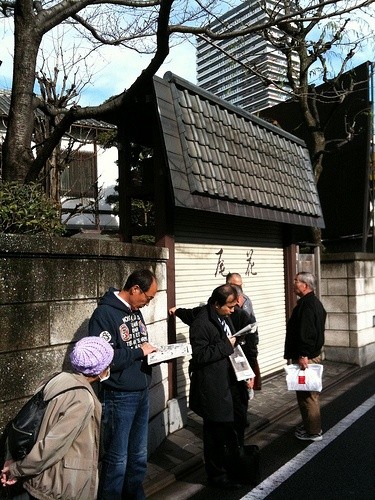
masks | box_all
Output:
[100,366,111,382]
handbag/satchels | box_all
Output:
[283,364,323,392]
[222,425,260,486]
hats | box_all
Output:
[70,336,114,375]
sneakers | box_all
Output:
[295,431,323,441]
[296,424,323,435]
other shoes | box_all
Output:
[208,464,241,489]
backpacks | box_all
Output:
[7,370,92,462]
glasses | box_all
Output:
[107,363,115,368]
[140,288,152,302]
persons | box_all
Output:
[226,272,256,329]
[169,284,261,455]
[88,269,158,500]
[188,284,256,487]
[0,336,114,500]
[283,272,327,441]
